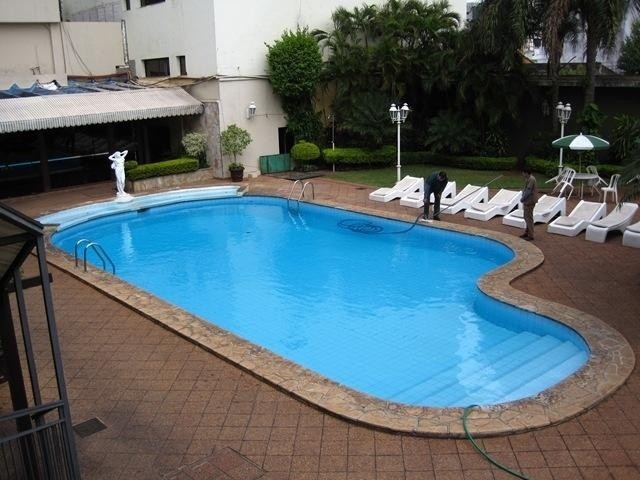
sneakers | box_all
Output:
[422,214,440,220]
[519,233,534,241]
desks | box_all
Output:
[566,173,599,199]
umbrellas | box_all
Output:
[552,130,610,200]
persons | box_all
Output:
[520,166,539,241]
[107,148,132,197]
[421,171,450,223]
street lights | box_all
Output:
[388,102,410,167]
[555,101,572,167]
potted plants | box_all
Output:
[218,124,252,181]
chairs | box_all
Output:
[544,166,568,195]
[586,166,608,196]
[503,194,567,228]
[370,175,424,204]
[601,173,620,204]
[464,188,524,222]
[553,167,576,200]
[586,202,638,243]
[433,184,489,215]
[546,199,607,237]
[622,221,640,248]
[400,181,456,208]
